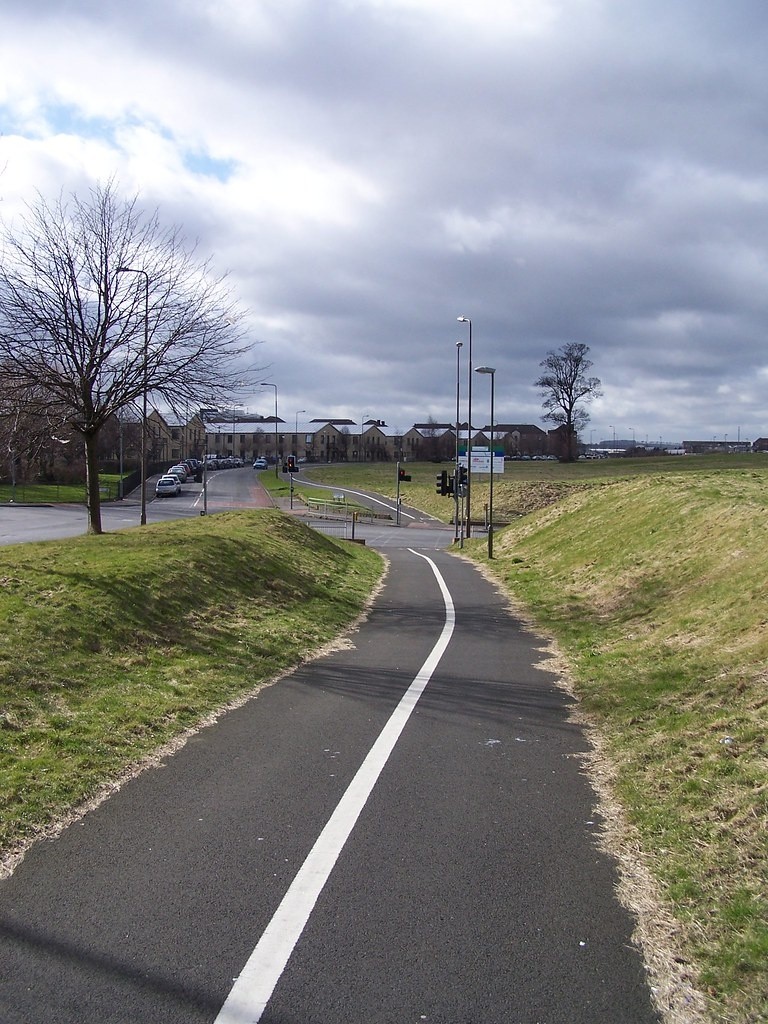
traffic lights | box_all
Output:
[435,470,448,497]
[288,456,300,473]
[398,469,411,482]
[457,465,468,485]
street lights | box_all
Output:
[456,316,472,539]
[725,434,727,451]
[115,265,148,525]
[232,403,244,458]
[629,427,635,448]
[609,426,615,449]
[295,410,306,463]
[455,341,464,538]
[259,383,279,479]
[590,429,596,444]
[361,414,369,434]
[474,366,496,559]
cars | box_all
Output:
[504,454,557,461]
[578,452,610,460]
[155,454,276,498]
[253,459,268,470]
[298,456,308,463]
